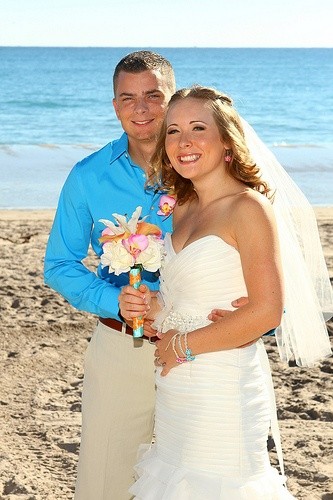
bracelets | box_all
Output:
[165,333,195,363]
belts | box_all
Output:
[99,317,160,344]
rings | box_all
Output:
[157,357,163,366]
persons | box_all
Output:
[44,50,285,500]
[119,87,333,500]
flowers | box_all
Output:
[98,206,166,338]
[157,192,178,222]
